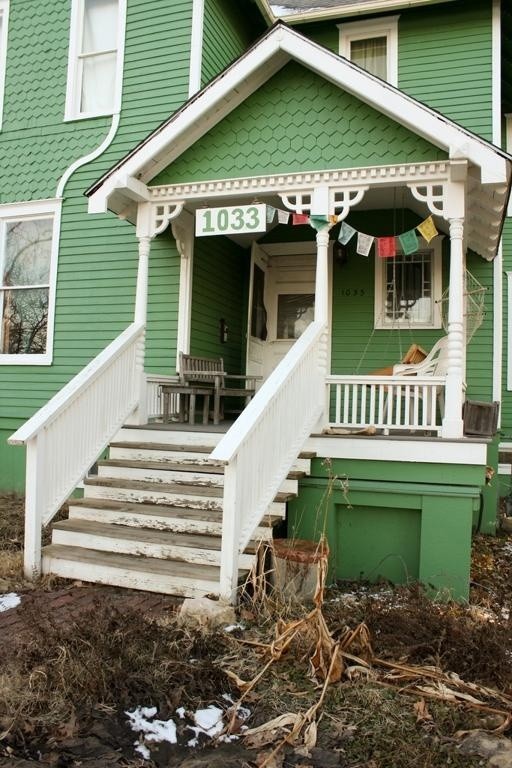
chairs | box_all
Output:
[179,350,262,427]
[382,335,446,435]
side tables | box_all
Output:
[159,384,214,426]
